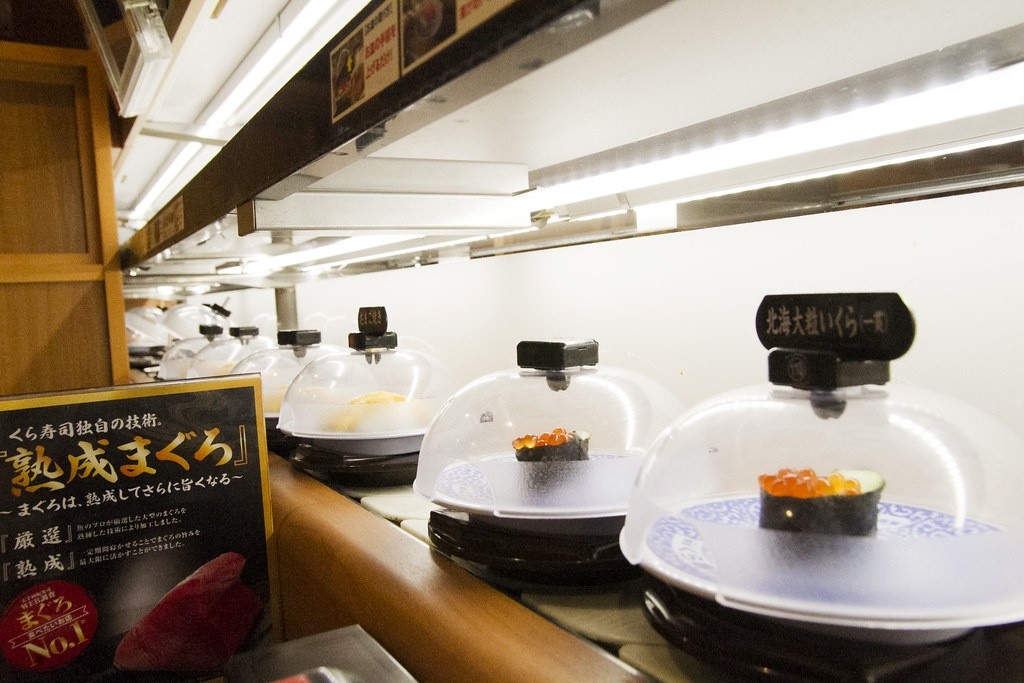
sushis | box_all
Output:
[513,431,590,464]
[761,468,885,533]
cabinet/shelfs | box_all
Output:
[116,0,1024,683]
